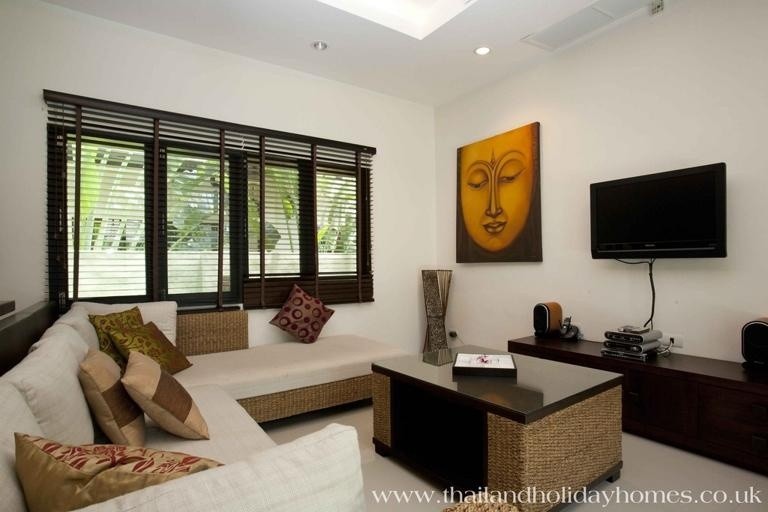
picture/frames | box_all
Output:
[456,121,544,264]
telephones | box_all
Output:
[557,316,579,342]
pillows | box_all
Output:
[268,284,336,343]
[78,346,147,446]
[87,306,146,371]
[119,349,210,440]
[13,432,224,511]
[106,321,191,377]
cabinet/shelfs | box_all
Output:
[506,334,768,477]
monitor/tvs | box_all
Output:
[589,162,728,259]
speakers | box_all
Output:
[533,301,562,338]
[740,317,768,370]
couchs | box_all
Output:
[0,301,396,512]
